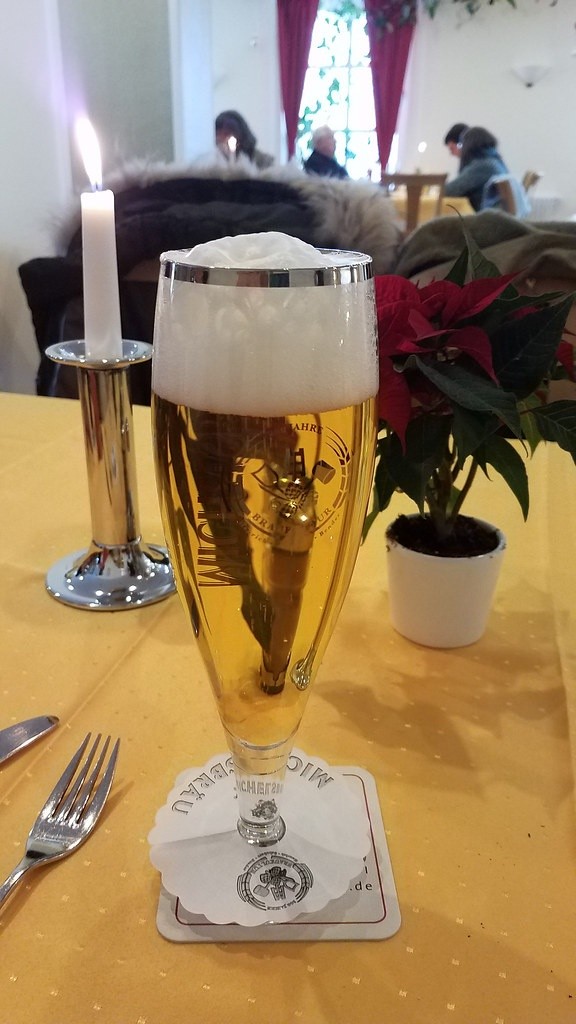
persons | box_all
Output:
[214,108,274,171]
[303,126,354,182]
[441,126,532,220]
[443,122,473,155]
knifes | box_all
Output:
[0,714,60,763]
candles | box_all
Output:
[78,184,131,365]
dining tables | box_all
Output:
[0,383,576,1022]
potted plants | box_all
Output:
[357,199,576,653]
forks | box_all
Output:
[0,732,120,911]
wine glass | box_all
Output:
[145,247,381,925]
